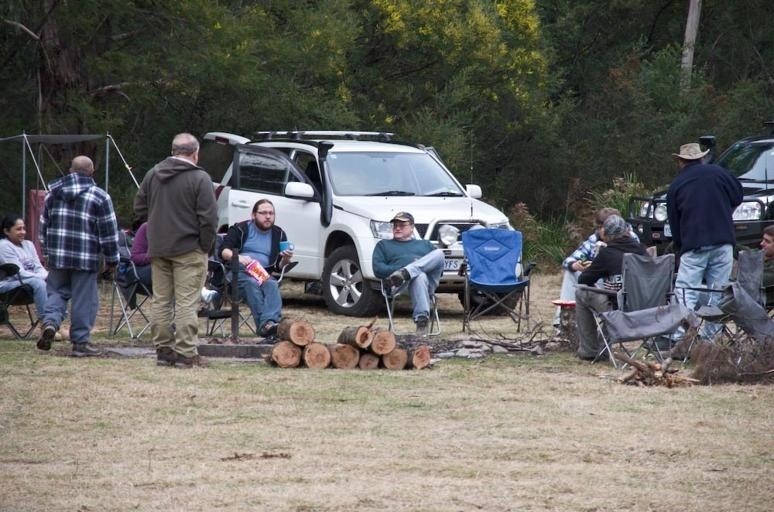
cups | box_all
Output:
[280,241,295,253]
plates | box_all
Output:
[550,299,576,308]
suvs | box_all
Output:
[624,121,774,261]
[196,124,524,318]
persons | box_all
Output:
[0,214,70,342]
[130,221,220,305]
[219,198,295,333]
[553,206,774,360]
[370,210,447,335]
[35,155,122,357]
[133,131,217,369]
[664,142,744,344]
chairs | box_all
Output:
[205,232,299,339]
[108,231,153,339]
[0,263,42,341]
[196,260,231,338]
[380,278,442,338]
[677,251,765,366]
[462,228,530,332]
[574,254,676,369]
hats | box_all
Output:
[671,143,710,161]
[603,215,625,234]
[390,212,414,224]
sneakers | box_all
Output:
[157,350,209,369]
[416,316,428,339]
[72,342,102,356]
[202,287,218,304]
[659,346,690,359]
[383,271,404,290]
[37,325,62,349]
[259,321,279,337]
